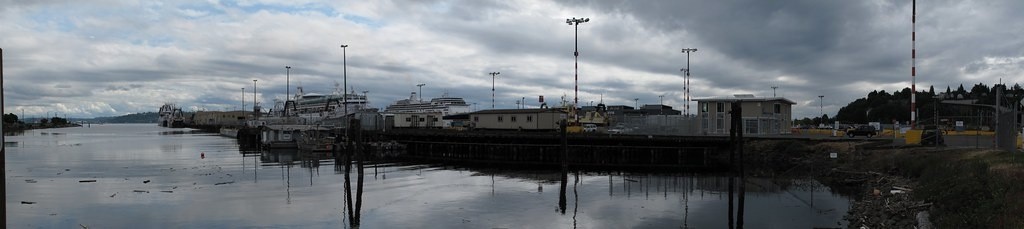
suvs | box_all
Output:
[847,125,877,138]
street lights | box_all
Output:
[252,78,258,113]
[817,95,824,123]
[417,83,425,111]
[681,48,697,118]
[659,94,665,115]
[679,66,688,116]
[285,66,291,115]
[489,71,500,109]
[566,16,589,110]
[771,85,778,98]
[241,87,247,113]
[340,43,349,118]
[634,97,639,113]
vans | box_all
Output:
[583,124,598,133]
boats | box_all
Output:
[158,78,379,154]
